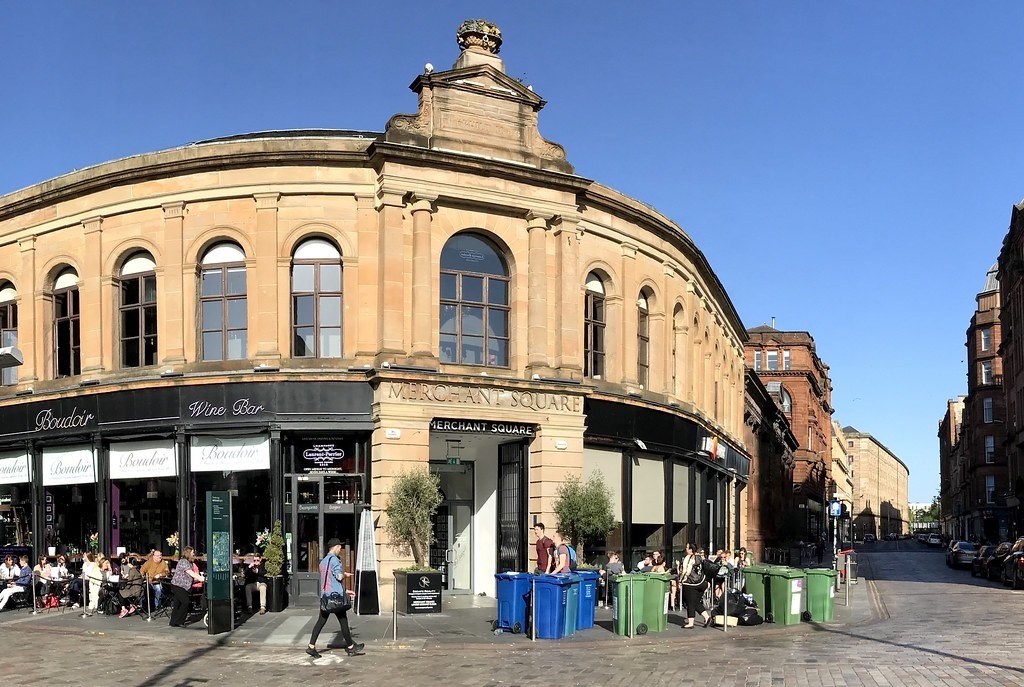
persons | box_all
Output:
[592,540,751,629]
[305,538,365,657]
[0,547,267,628]
[815,535,828,566]
[534,523,556,575]
[550,529,572,573]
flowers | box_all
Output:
[256,527,272,548]
[89,532,99,549]
[166,531,179,547]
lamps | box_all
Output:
[365,368,376,378]
[633,438,647,450]
[698,451,710,457]
[442,304,470,320]
[531,373,541,380]
[424,62,434,75]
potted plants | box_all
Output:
[45,528,57,556]
[382,465,444,617]
[549,465,624,609]
[264,520,285,612]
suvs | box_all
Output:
[863,534,874,544]
[986,541,1013,579]
[971,545,998,577]
[1000,536,1024,590]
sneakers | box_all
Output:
[306,647,321,658]
[348,642,364,655]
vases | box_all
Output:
[92,548,99,555]
[174,545,179,557]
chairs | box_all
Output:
[0,558,264,624]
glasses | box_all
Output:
[739,553,745,555]
[721,556,726,559]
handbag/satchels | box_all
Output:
[46,593,60,608]
[702,559,720,581]
[321,593,352,612]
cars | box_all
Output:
[883,533,979,569]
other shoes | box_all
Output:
[129,605,137,613]
[260,608,265,615]
[119,609,128,618]
[704,618,712,628]
[681,623,694,629]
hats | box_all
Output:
[328,538,345,547]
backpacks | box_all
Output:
[560,543,577,570]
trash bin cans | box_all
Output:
[556,572,584,637]
[640,571,675,632]
[803,567,838,623]
[492,572,533,634]
[609,573,651,636]
[743,565,771,623]
[766,565,806,626]
[570,569,601,631]
[734,550,753,565]
[528,573,573,639]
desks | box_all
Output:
[228,338,242,359]
